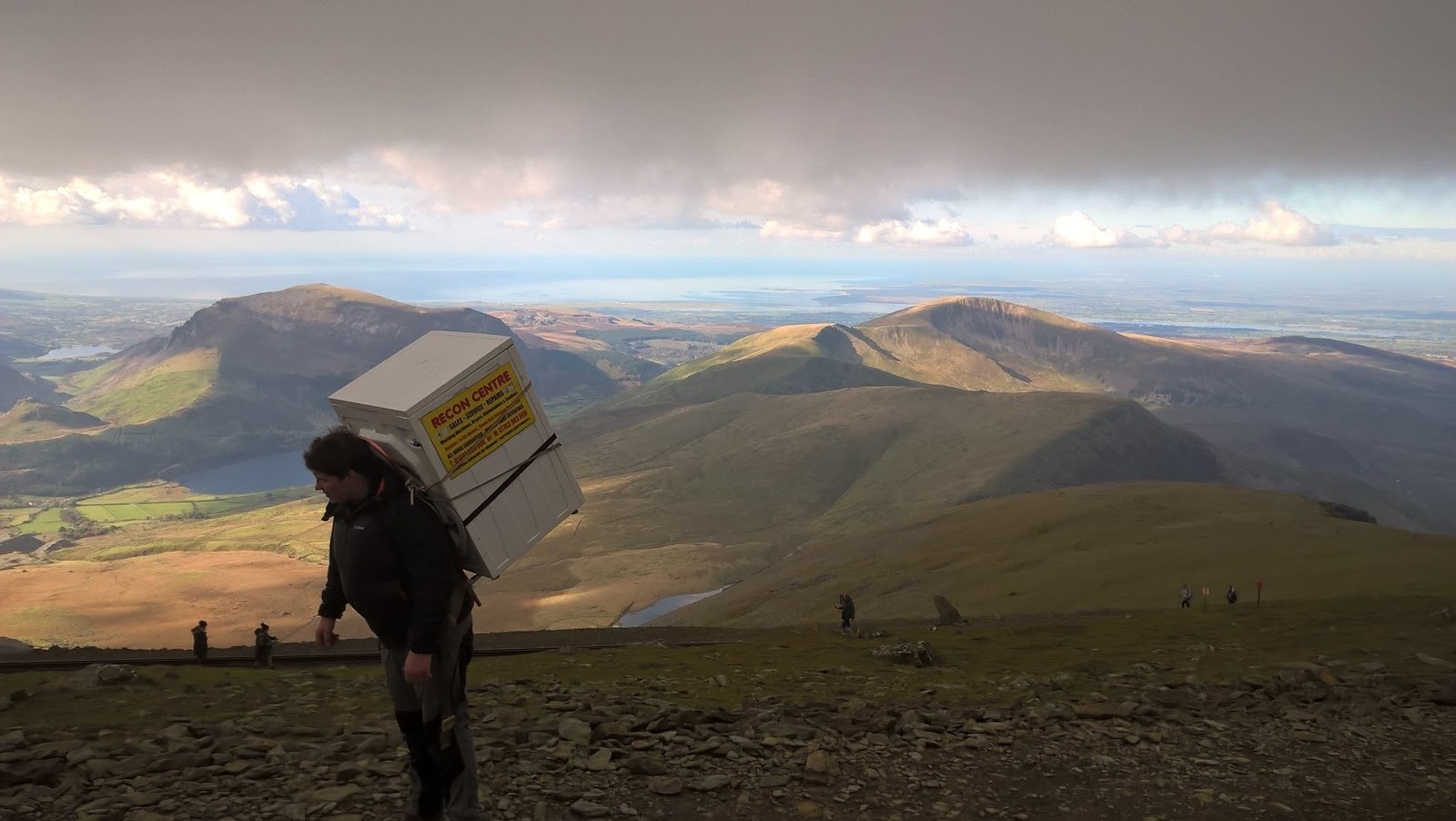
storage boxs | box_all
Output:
[330,329,586,582]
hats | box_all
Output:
[199,621,207,626]
[261,623,269,630]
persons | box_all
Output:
[254,623,279,669]
[925,593,968,632]
[1178,584,1192,609]
[835,594,854,629]
[301,426,482,821]
[191,620,208,665]
[1226,586,1237,604]
